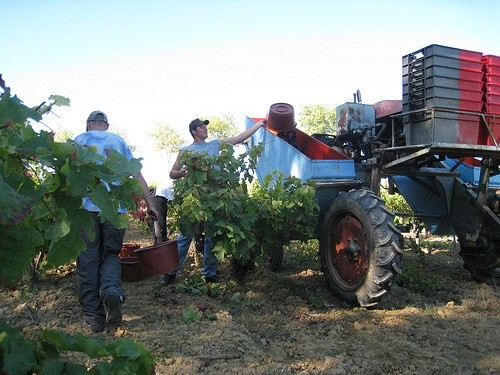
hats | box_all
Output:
[189,118,209,132]
[87,111,107,131]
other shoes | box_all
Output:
[159,276,174,287]
[104,293,122,326]
[206,275,223,283]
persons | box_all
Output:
[159,118,266,285]
[149,186,175,244]
[74,111,159,332]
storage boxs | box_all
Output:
[402,44,500,145]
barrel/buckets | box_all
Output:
[133,214,180,276]
[119,257,143,282]
[266,103,297,133]
[119,244,140,258]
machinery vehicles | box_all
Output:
[239,89,500,309]
